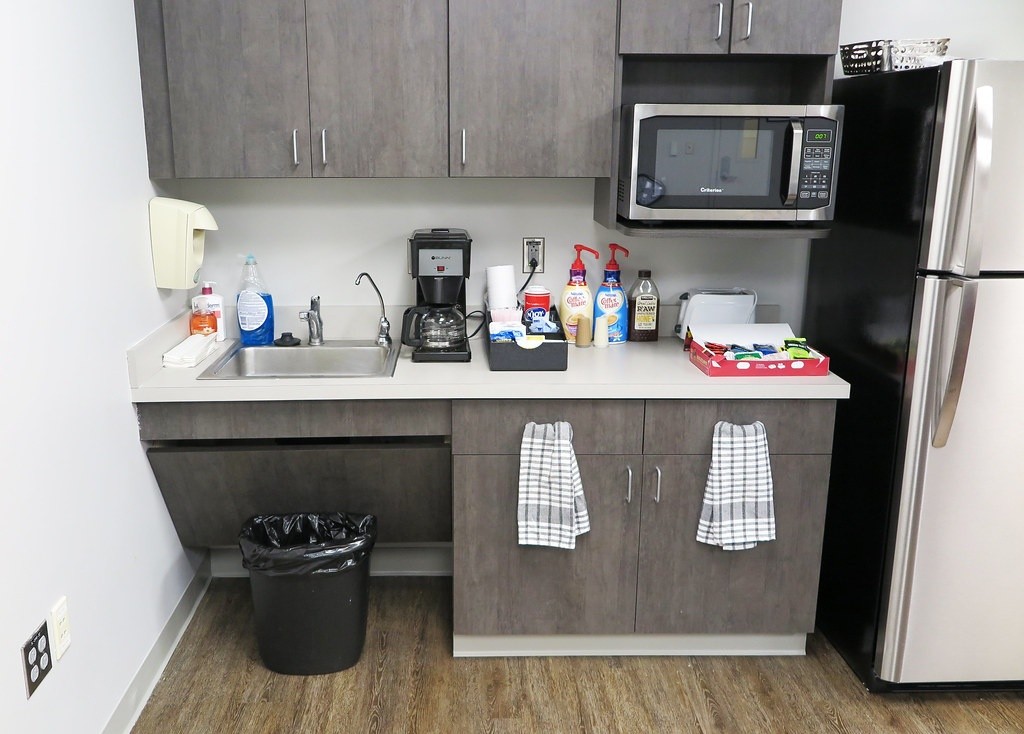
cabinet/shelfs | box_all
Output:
[450,0,623,178]
[161,0,448,179]
[619,0,843,105]
[451,398,837,654]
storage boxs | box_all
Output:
[683,324,829,377]
[486,301,568,373]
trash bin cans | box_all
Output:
[238,514,378,676]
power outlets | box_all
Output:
[50,596,71,660]
[21,619,53,700]
[522,237,545,273]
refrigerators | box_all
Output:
[800,55,1024,695]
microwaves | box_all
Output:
[619,104,848,229]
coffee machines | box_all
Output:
[401,228,472,363]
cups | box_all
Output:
[575,318,592,348]
[593,317,609,349]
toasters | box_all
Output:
[674,286,757,340]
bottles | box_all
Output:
[236,255,275,347]
[627,269,661,342]
